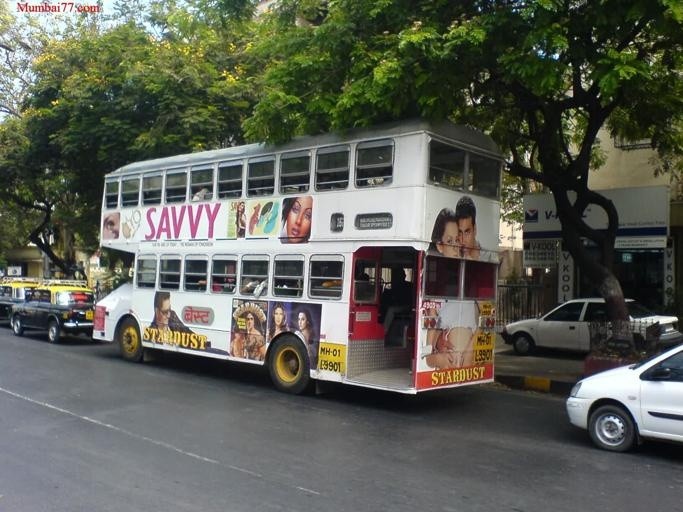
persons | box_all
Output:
[292,306,318,357]
[233,202,246,237]
[106,213,120,235]
[423,300,485,374]
[233,312,265,362]
[382,266,413,341]
[269,303,291,349]
[280,196,311,243]
[191,176,213,201]
[146,289,229,356]
[197,259,300,299]
[456,195,498,264]
[428,208,460,257]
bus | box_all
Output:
[88,114,509,405]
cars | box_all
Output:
[498,292,682,359]
[563,341,682,455]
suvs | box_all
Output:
[0,274,37,322]
[9,279,96,344]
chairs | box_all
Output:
[380,281,418,347]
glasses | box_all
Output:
[161,305,172,315]
